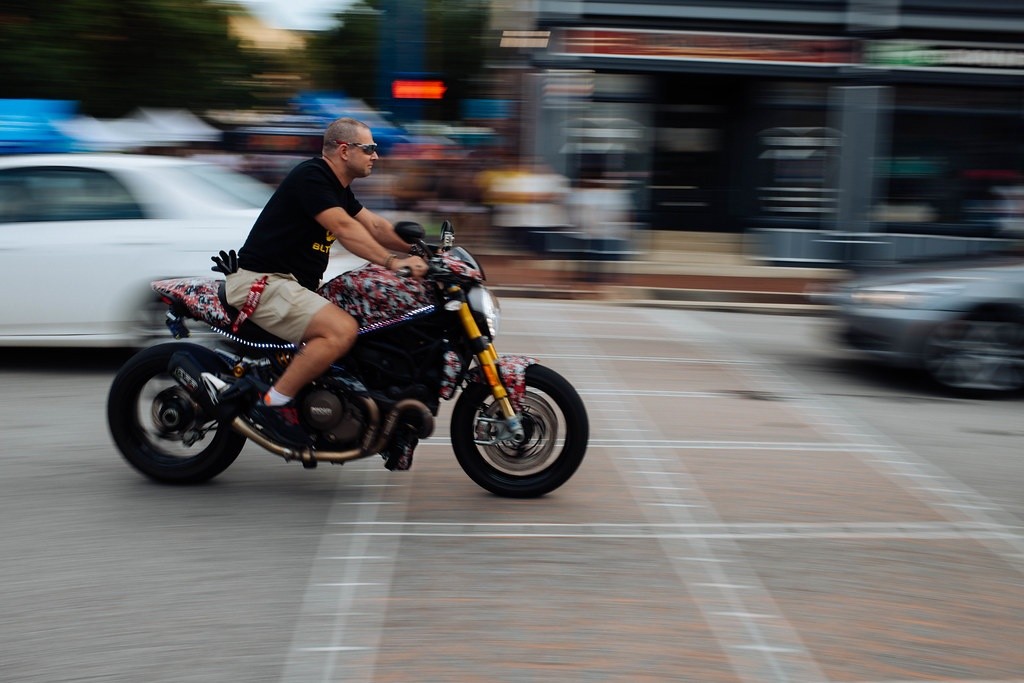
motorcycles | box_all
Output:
[107,219,589,497]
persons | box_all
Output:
[227,118,429,455]
[475,154,632,261]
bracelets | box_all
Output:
[385,255,396,269]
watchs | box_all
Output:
[411,244,418,253]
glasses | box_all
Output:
[335,141,378,155]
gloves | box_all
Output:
[211,250,239,275]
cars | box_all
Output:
[830,252,1024,397]
[0,153,369,347]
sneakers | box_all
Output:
[248,395,307,449]
